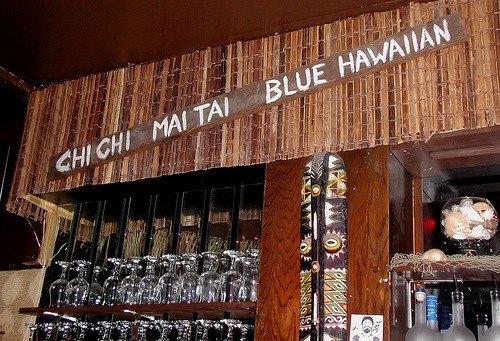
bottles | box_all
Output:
[404,288,436,341]
[426,289,443,341]
[441,291,477,341]
[478,289,500,341]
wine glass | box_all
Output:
[49,261,70,307]
[24,317,254,341]
[63,248,260,303]
